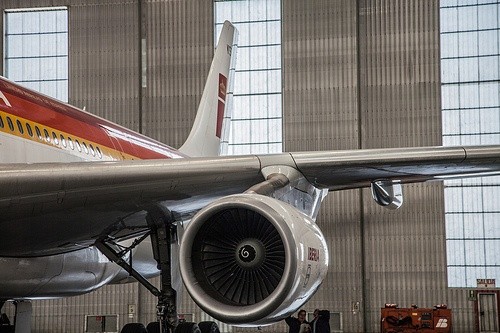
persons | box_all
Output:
[284,309,311,333]
[309,308,332,332]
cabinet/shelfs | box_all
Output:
[381,308,452,333]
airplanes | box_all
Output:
[1,20,500,332]
